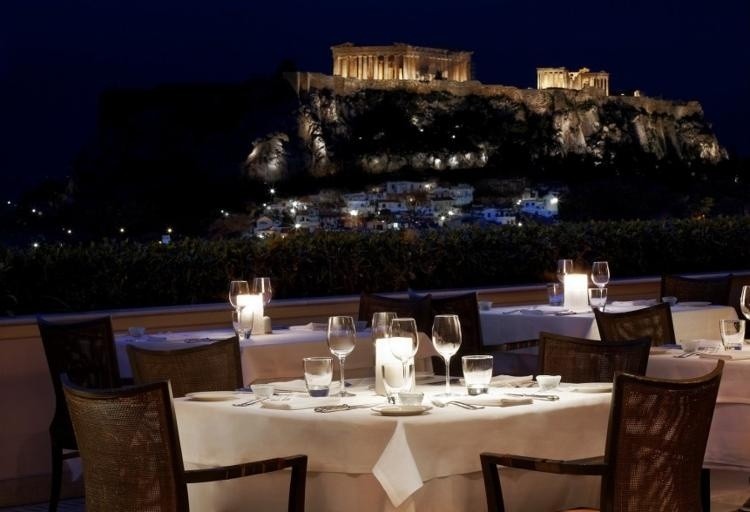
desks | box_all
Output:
[116,321,439,376]
[480,297,648,373]
[168,375,618,512]
[650,340,750,476]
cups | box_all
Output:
[251,383,274,398]
[536,374,561,391]
[719,319,745,351]
[381,364,414,404]
[740,285,750,320]
[461,354,493,397]
[303,357,332,396]
[228,276,273,338]
[544,259,611,315]
[663,296,677,306]
[397,391,424,405]
[372,313,399,348]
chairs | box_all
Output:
[659,271,737,304]
[39,314,132,509]
[594,301,679,347]
[125,335,245,395]
[60,374,308,512]
[535,332,653,382]
[405,288,494,376]
[479,359,732,511]
[355,292,434,331]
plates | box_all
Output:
[186,390,240,399]
[678,302,712,306]
[372,404,434,415]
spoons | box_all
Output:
[432,397,484,409]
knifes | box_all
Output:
[316,403,376,414]
[232,397,268,406]
[507,393,559,400]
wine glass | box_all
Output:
[387,319,420,378]
[327,315,358,397]
[432,315,463,397]
